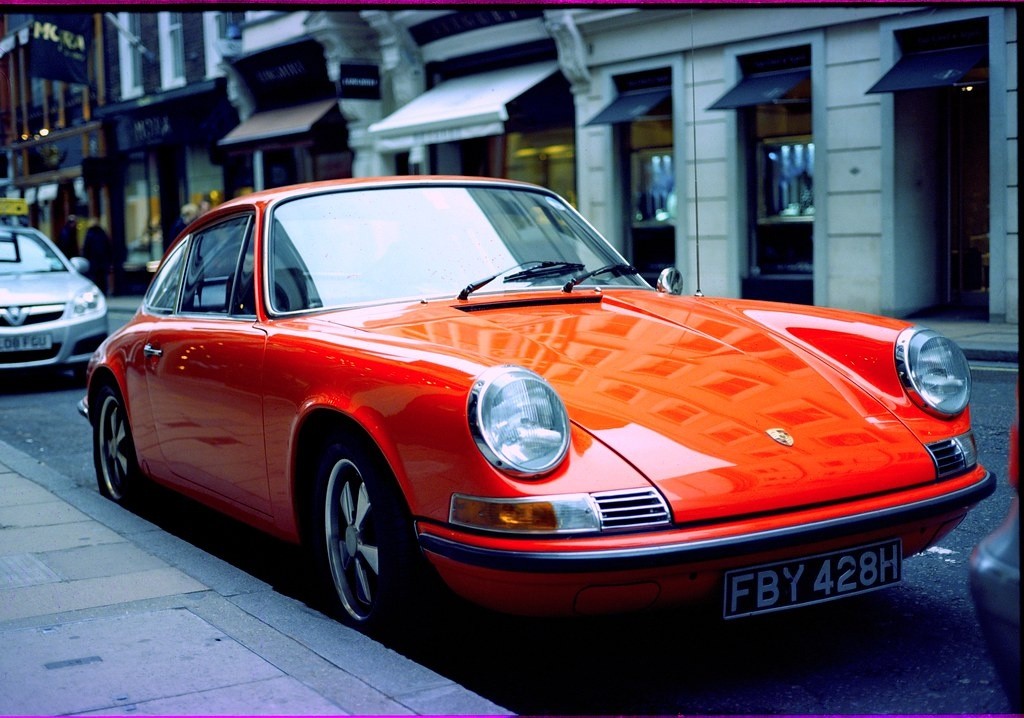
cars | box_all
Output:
[0,225,109,392]
[75,174,996,647]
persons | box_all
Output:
[59,214,110,298]
